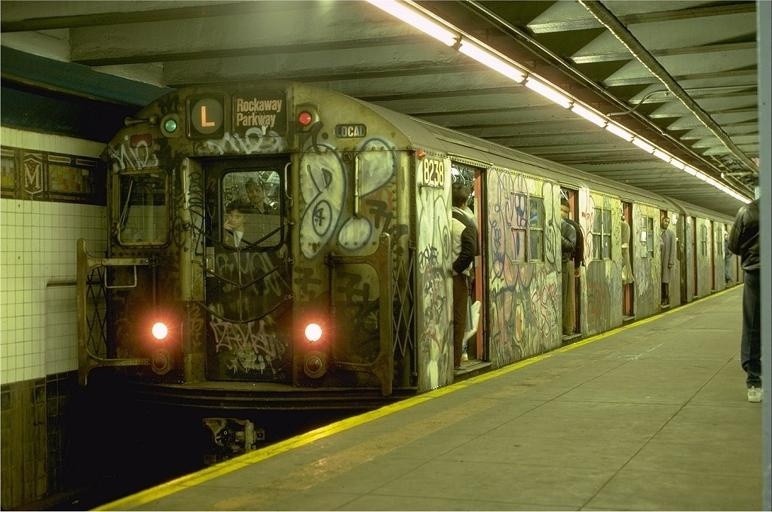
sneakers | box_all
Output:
[747,386,762,402]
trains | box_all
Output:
[75,77,743,466]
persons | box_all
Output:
[659,216,675,308]
[222,198,266,247]
[561,198,576,338]
[725,232,733,288]
[245,179,280,247]
[451,182,480,370]
[727,195,764,401]
[563,216,583,330]
